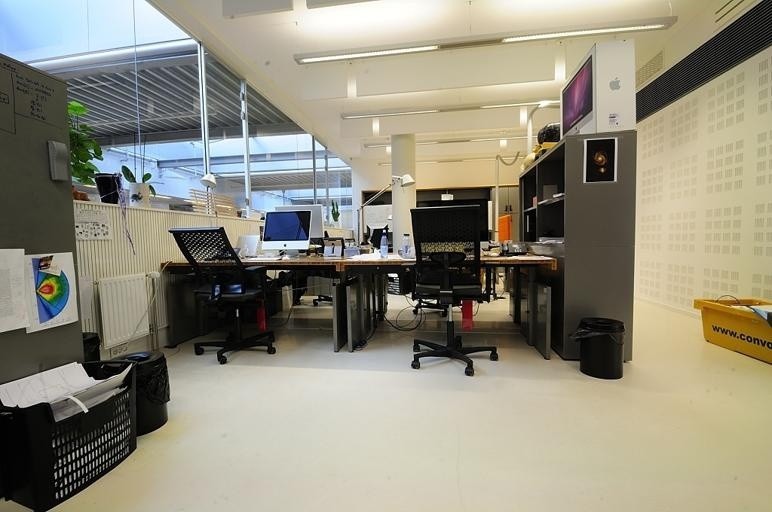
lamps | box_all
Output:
[200,173,223,227]
[352,174,415,253]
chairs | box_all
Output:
[167,226,282,366]
[410,205,500,379]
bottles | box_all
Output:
[380,229,388,260]
[402,233,411,260]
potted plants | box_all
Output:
[329,200,341,227]
[120,165,167,207]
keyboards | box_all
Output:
[248,256,281,261]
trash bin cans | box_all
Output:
[104,350,171,439]
[567,316,626,380]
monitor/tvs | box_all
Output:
[274,204,325,249]
[261,210,313,261]
[559,44,595,139]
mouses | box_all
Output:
[283,255,290,261]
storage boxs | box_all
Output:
[693,296,771,365]
[0,359,137,511]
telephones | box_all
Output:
[502,242,528,257]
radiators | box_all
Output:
[96,273,152,348]
[153,268,166,328]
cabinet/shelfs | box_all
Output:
[520,129,635,362]
[493,187,519,243]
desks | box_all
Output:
[346,248,558,362]
[162,250,344,352]
